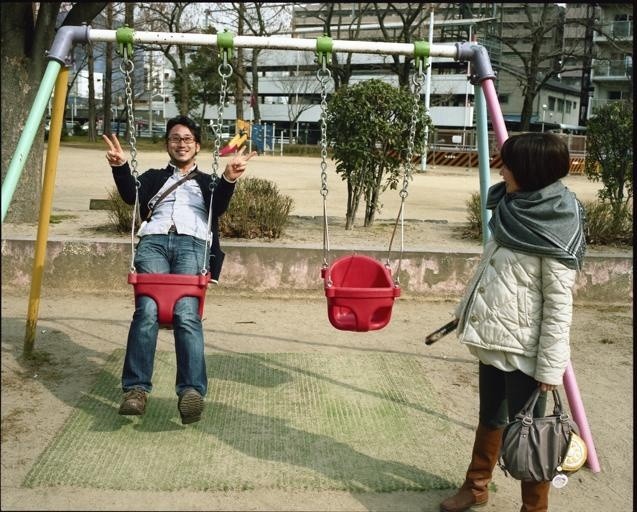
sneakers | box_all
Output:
[178,388,205,426]
[118,380,150,416]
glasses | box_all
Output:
[169,136,195,144]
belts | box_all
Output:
[168,225,179,234]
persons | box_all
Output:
[102,116,256,425]
[440,133,585,512]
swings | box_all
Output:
[311,51,429,333]
[115,41,232,331]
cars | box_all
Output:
[44,119,307,143]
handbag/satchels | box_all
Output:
[500,416,582,481]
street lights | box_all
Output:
[540,104,547,133]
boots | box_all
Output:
[440,422,509,511]
[520,478,549,512]
